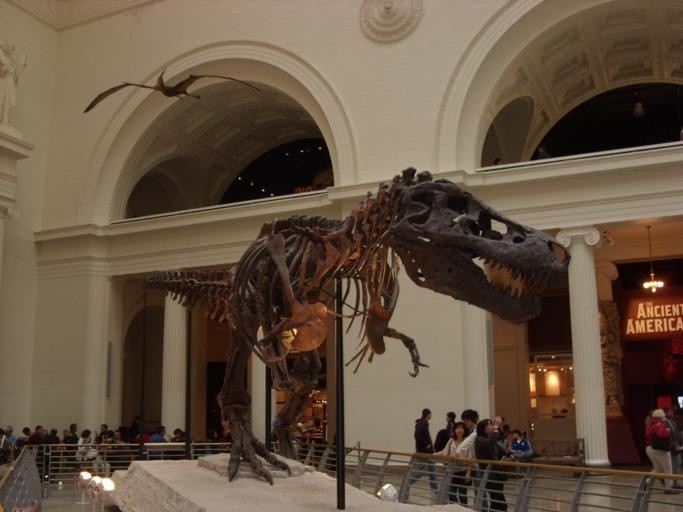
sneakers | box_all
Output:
[664,484,683,494]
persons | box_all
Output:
[642,406,683,495]
[404,406,535,512]
[0,414,232,487]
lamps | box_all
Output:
[643,225,664,293]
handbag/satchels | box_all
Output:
[651,422,669,451]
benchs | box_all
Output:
[529,438,587,478]
